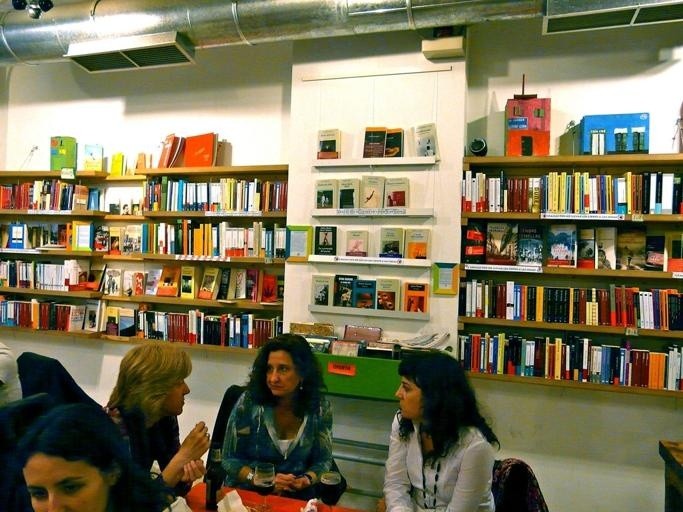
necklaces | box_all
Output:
[418,422,441,509]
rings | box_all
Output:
[203,432,209,439]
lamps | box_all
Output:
[11,0,54,18]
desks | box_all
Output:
[658,439,683,512]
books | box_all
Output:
[141,218,285,259]
[363,127,404,157]
[109,225,143,254]
[314,226,337,256]
[0,294,108,332]
[105,306,137,337]
[457,333,681,391]
[137,309,283,349]
[314,176,410,210]
[461,219,681,272]
[380,228,406,258]
[145,263,285,302]
[310,274,429,314]
[461,170,681,214]
[290,323,454,360]
[157,134,232,167]
[415,123,442,162]
[0,255,107,292]
[458,279,681,331]
[0,178,106,211]
[404,229,430,259]
[105,269,144,296]
[49,136,152,177]
[345,230,369,257]
[318,129,342,158]
[144,175,288,212]
[0,219,108,252]
[107,199,142,215]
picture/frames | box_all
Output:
[431,262,458,296]
[285,225,312,262]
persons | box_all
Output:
[380,344,501,511]
[0,342,22,413]
[219,333,335,505]
[0,401,167,511]
[99,339,211,509]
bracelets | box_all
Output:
[245,469,256,484]
[296,472,312,486]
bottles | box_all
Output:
[206,442,226,510]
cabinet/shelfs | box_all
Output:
[308,154,435,320]
[133,163,289,354]
[96,174,147,342]
[0,170,107,339]
[308,352,406,400]
[457,151,683,397]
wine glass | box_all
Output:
[320,471,342,512]
[255,463,276,511]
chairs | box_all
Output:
[203,383,347,506]
[0,351,103,512]
[487,454,549,512]
